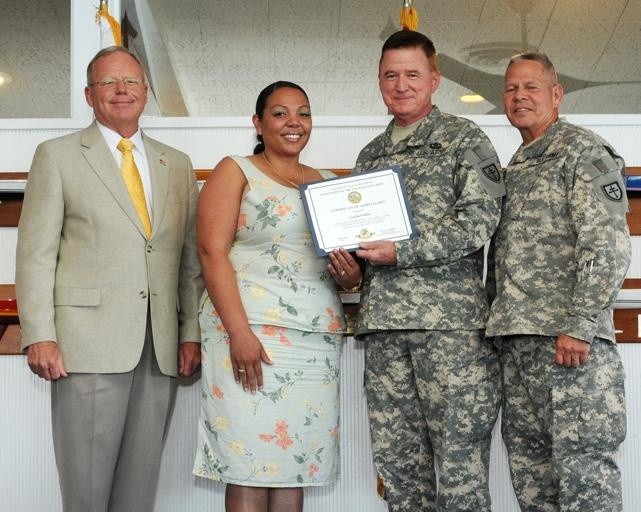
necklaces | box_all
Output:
[262,150,304,189]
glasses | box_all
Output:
[90,80,144,87]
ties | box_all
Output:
[117,139,152,239]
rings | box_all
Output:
[341,270,345,275]
[238,369,245,372]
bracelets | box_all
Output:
[341,279,362,294]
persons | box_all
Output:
[192,79,364,512]
[14,43,210,512]
[352,28,509,512]
[484,51,633,512]
